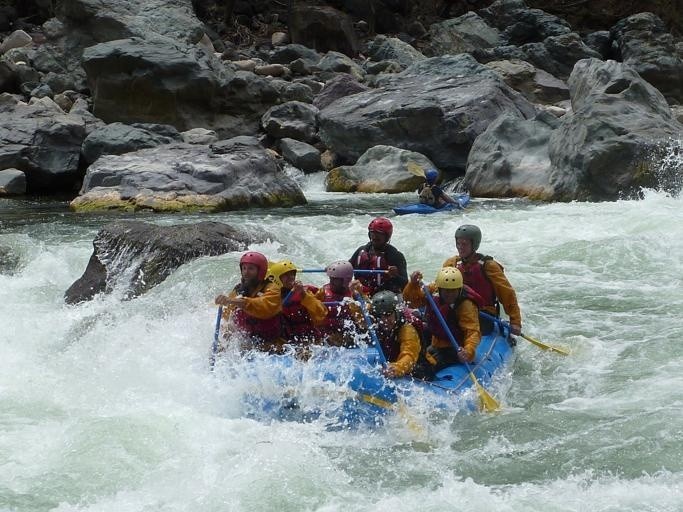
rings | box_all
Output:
[462,356,464,359]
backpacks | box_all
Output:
[417,182,436,206]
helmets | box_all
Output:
[424,168,438,179]
[368,218,393,244]
[369,290,399,315]
[324,260,353,289]
[267,259,298,290]
[455,224,482,253]
[434,265,464,291]
[239,251,267,283]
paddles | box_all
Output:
[354,290,421,435]
[266,260,390,274]
[479,309,568,356]
[418,278,499,413]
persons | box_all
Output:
[211,251,281,365]
[441,223,522,337]
[347,214,408,300]
[267,259,329,364]
[363,289,427,383]
[402,266,482,375]
[319,260,368,351]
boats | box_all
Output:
[235,315,514,439]
[394,191,471,216]
